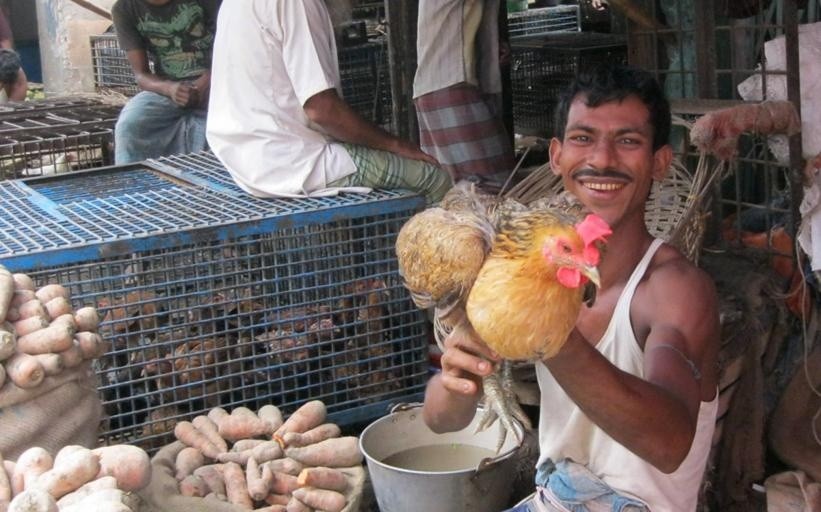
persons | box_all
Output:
[112,0,217,170]
[204,0,450,203]
[421,66,726,512]
[413,0,512,192]
[0,49,27,104]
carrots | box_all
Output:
[1,263,364,512]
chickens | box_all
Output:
[20,151,76,177]
[73,236,427,455]
[392,169,618,454]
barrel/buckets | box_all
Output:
[359,401,524,512]
